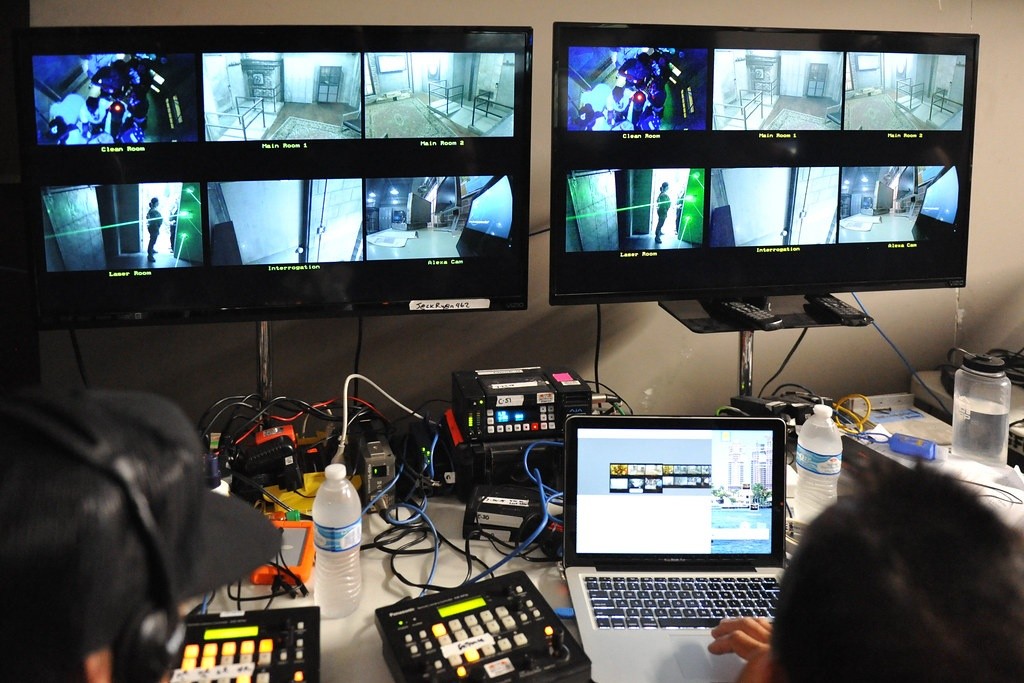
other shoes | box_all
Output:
[655,237,662,242]
[660,232,665,235]
[153,249,158,252]
[148,256,155,261]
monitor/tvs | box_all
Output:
[12,23,981,329]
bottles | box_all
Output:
[312,463,364,619]
[793,404,843,525]
[948,348,1012,468]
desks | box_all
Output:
[911,371,1024,460]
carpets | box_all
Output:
[268,117,342,140]
[765,109,824,130]
[365,96,457,139]
[844,94,917,130]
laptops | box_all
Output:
[561,415,790,683]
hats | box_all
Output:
[0,390,283,669]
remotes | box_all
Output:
[804,294,874,327]
[698,300,783,332]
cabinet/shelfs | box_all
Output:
[317,66,342,104]
[804,62,828,98]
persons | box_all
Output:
[709,468,1024,683]
[169,196,179,252]
[0,390,285,682]
[147,197,163,261]
[655,182,671,243]
[676,184,686,233]
[578,48,673,130]
[49,58,152,144]
